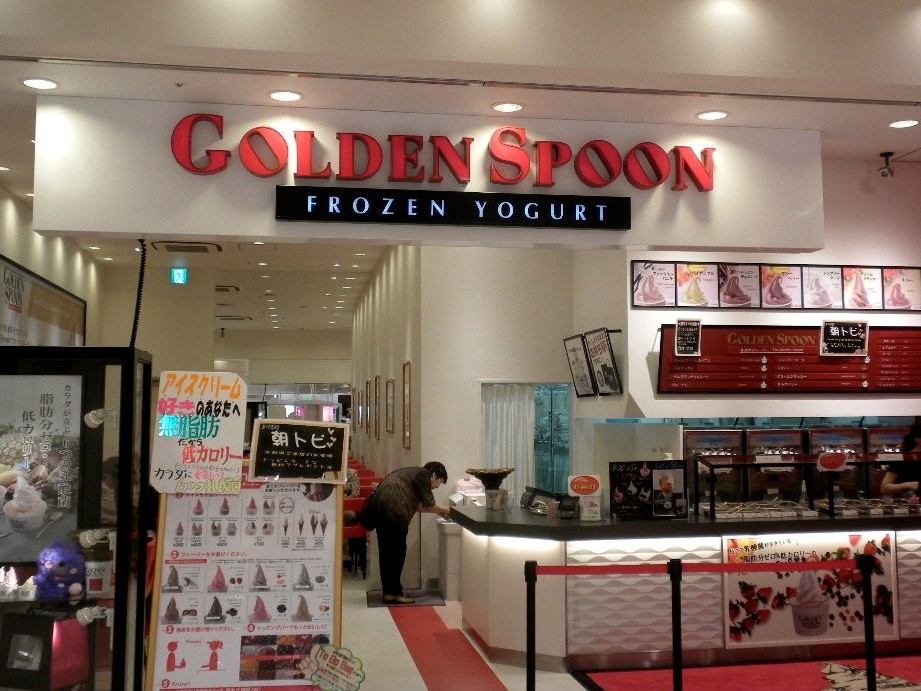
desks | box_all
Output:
[1,598,96,691]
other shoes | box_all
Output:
[383,593,415,604]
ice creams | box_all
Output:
[633,275,666,306]
[2,475,48,532]
[886,280,911,309]
[762,276,793,308]
[806,277,832,308]
[847,271,870,309]
[720,276,752,307]
[789,556,830,637]
[614,460,676,509]
[162,496,328,623]
[678,277,709,306]
[0,566,37,601]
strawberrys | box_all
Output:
[729,580,798,644]
[818,534,891,591]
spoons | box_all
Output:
[31,512,64,540]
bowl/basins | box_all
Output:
[4,498,49,531]
[789,599,831,637]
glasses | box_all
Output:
[436,472,444,486]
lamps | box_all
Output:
[76,604,113,630]
[78,529,116,553]
[83,407,120,433]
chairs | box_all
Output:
[342,455,386,578]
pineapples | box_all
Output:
[0,420,81,469]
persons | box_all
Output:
[879,422,921,495]
[358,460,450,605]
[342,466,361,496]
[101,457,123,525]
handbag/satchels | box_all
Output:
[360,492,382,531]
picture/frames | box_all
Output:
[402,360,412,449]
[356,390,363,428]
[374,375,380,439]
[365,381,371,438]
[351,389,357,433]
[385,380,395,433]
